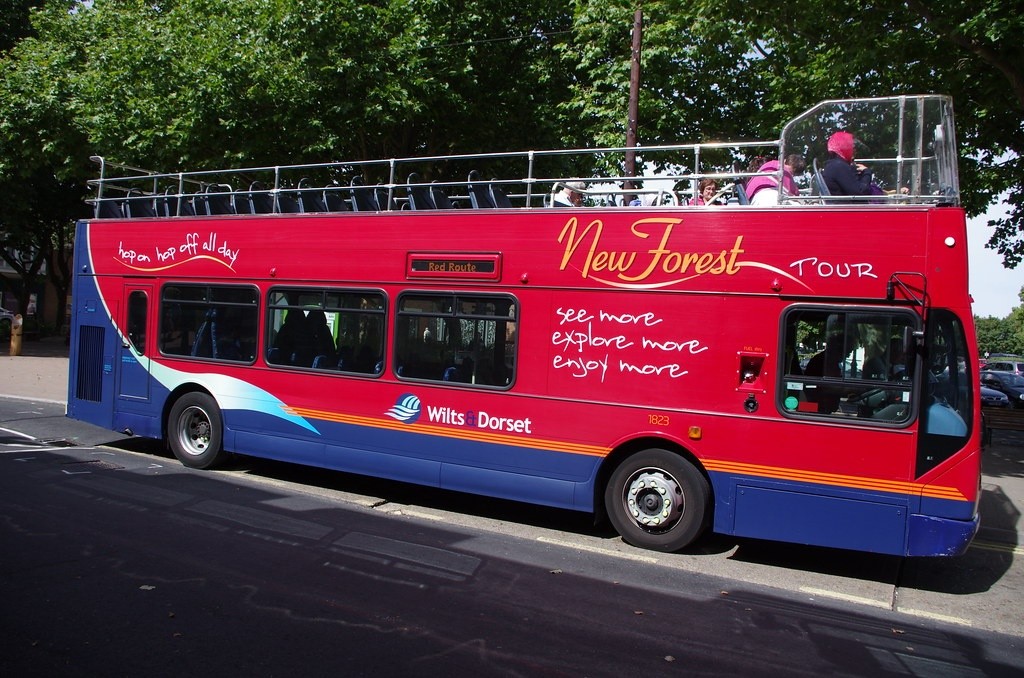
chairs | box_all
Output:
[99,155,844,219]
[126,289,514,388]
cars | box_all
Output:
[797,353,1024,409]
[0,272,71,345]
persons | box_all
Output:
[554,177,586,206]
[747,154,804,207]
[275,311,336,366]
[824,132,909,204]
[803,334,858,414]
[689,179,722,205]
[863,339,905,379]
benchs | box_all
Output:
[980,406,1024,448]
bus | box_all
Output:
[64,94,983,557]
